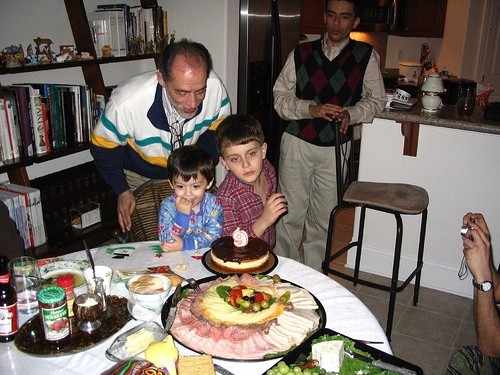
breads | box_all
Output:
[177,355,216,375]
[128,276,165,294]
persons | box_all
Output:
[158,145,224,252]
[129,29,175,56]
[462,212,500,357]
[216,113,287,250]
[273,0,388,270]
[89,37,232,233]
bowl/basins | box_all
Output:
[125,273,173,309]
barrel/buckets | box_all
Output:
[91,4,130,57]
[137,0,167,55]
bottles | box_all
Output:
[73,294,100,333]
[0,256,18,342]
[93,278,107,312]
[57,274,76,319]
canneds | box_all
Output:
[36,286,70,344]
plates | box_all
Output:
[38,261,91,290]
[99,355,172,375]
[259,328,424,375]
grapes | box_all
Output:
[242,300,269,310]
[273,274,280,283]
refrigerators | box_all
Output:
[237,0,304,177]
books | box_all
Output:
[0,83,105,159]
[90,4,167,57]
[0,182,45,248]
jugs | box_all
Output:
[420,71,447,93]
[421,91,444,113]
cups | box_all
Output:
[8,256,41,315]
[84,266,112,296]
[458,86,475,118]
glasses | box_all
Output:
[169,120,183,151]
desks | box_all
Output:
[0,240,393,375]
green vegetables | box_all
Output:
[215,285,276,313]
[263,333,404,375]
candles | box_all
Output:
[232,227,248,247]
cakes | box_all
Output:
[211,228,270,272]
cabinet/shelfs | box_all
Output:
[301,0,328,33]
[0,52,173,278]
[387,0,448,38]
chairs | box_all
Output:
[113,177,219,243]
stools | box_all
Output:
[322,118,429,343]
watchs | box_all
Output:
[472,280,493,292]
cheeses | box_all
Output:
[311,340,344,373]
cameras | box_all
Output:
[461,218,476,243]
